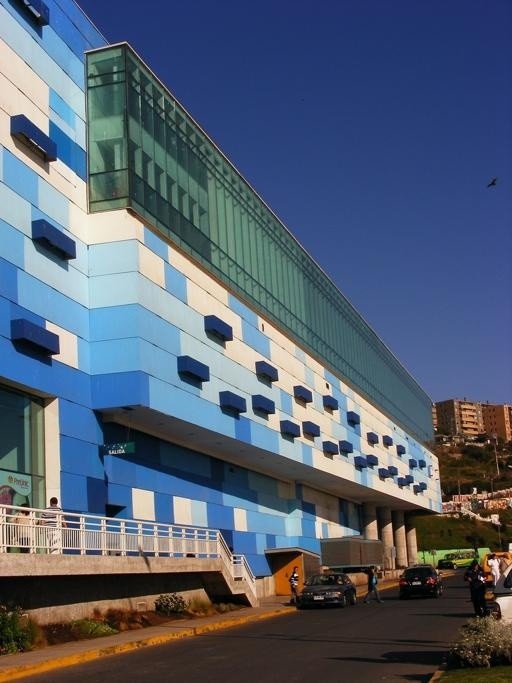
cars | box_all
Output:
[399,563,443,598]
[482,552,512,628]
[300,572,358,607]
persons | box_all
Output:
[11,503,35,554]
[488,553,501,587]
[464,560,488,617]
[289,565,300,605]
[363,565,385,603]
[41,497,68,555]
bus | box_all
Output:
[441,550,481,569]
